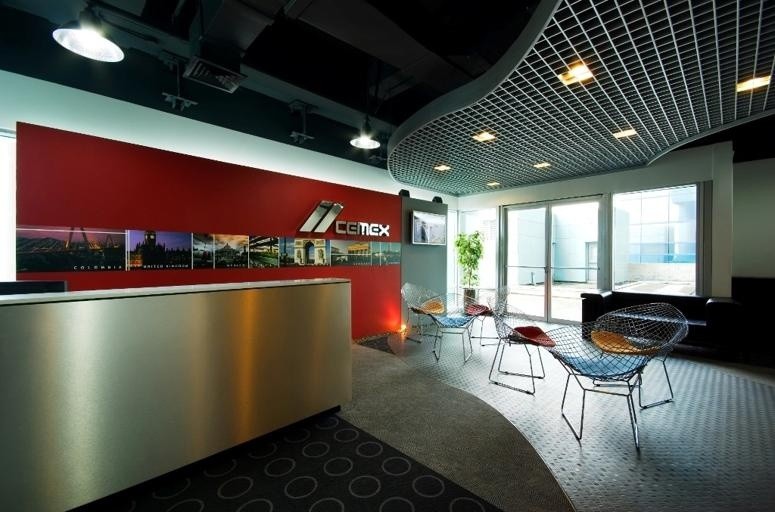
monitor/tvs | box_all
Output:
[0,280,69,295]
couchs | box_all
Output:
[578,288,735,364]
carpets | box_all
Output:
[65,344,573,512]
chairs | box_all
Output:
[705,276,775,368]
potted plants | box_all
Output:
[454,230,483,313]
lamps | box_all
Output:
[348,114,382,151]
[50,0,126,64]
